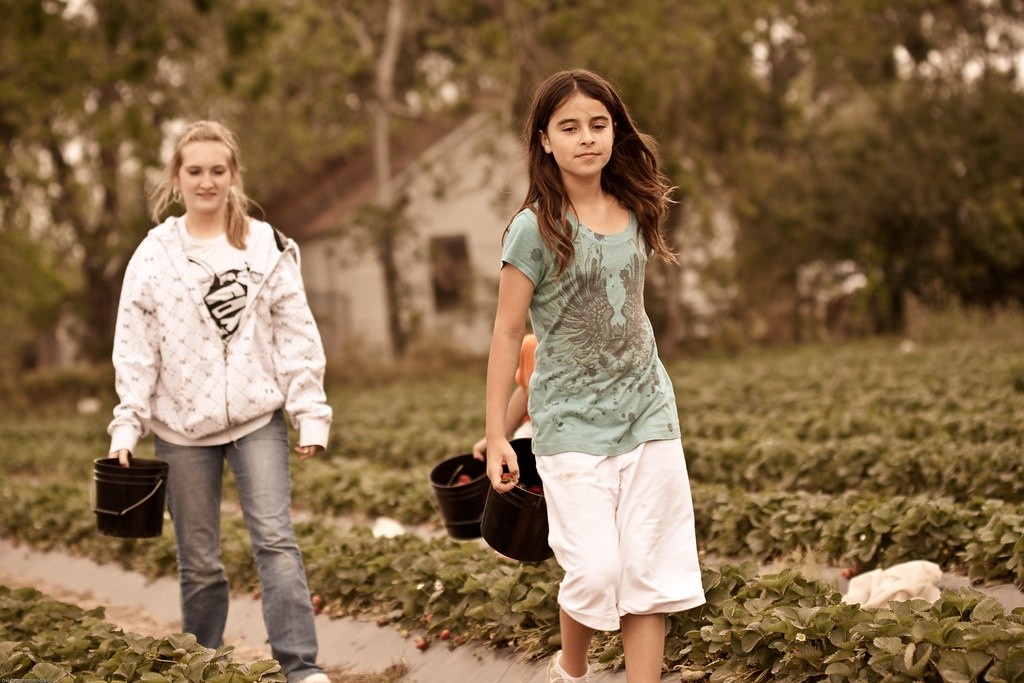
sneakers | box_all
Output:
[546,649,592,683]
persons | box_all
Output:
[480,70,707,682]
[106,121,335,680]
[469,331,541,460]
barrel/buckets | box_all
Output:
[431,456,491,540]
[480,437,554,563]
[90,455,168,538]
